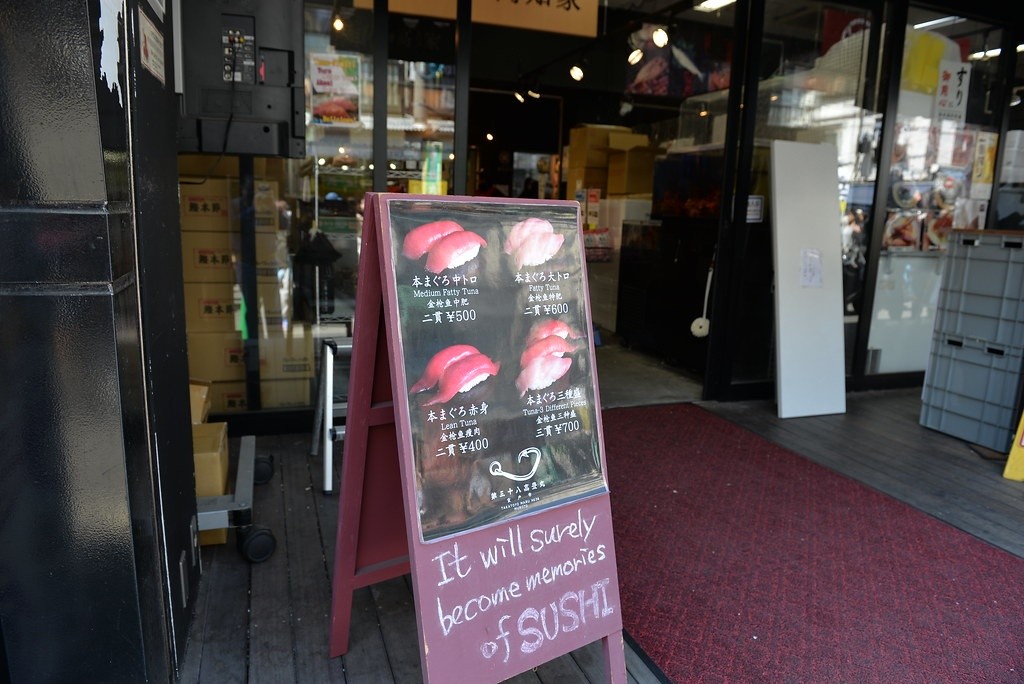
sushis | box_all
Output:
[409,344,500,406]
[514,319,578,396]
[503,216,565,271]
[311,97,358,120]
[402,219,486,274]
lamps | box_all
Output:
[528,80,543,99]
[651,25,680,48]
[514,82,531,102]
[620,42,643,65]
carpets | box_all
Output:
[602,400,1024,684]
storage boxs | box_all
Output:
[567,167,608,201]
[187,321,314,382]
[208,379,309,414]
[569,125,632,167]
[178,176,277,233]
[590,133,664,194]
[192,421,233,545]
[183,267,291,332]
[190,385,213,423]
[183,229,287,283]
